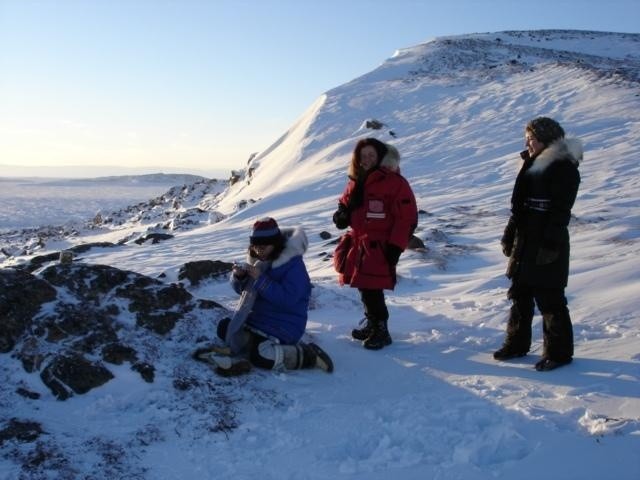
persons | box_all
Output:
[334,138,418,349]
[494,117,584,371]
[217,217,333,373]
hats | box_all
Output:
[529,116,564,144]
[249,216,283,246]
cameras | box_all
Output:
[336,209,351,229]
[234,263,247,271]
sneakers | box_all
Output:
[535,356,574,371]
[313,344,333,375]
[493,346,528,361]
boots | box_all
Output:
[351,311,392,350]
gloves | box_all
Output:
[333,203,350,229]
[386,245,402,266]
